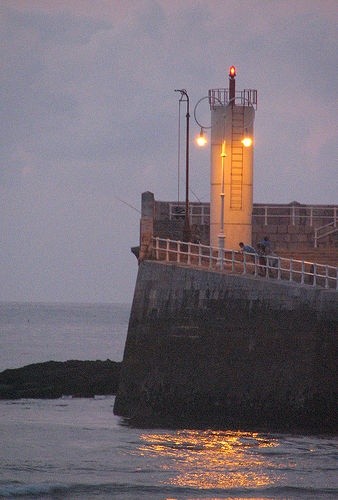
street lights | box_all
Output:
[193,96,256,274]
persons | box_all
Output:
[239,237,279,275]
